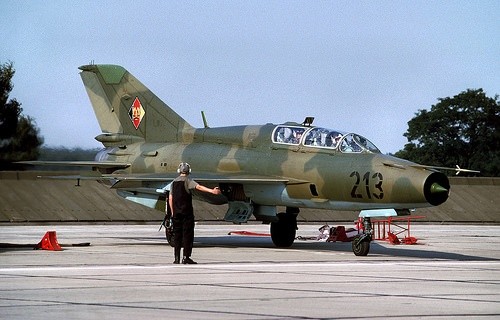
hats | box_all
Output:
[179,163,189,172]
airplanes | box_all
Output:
[14,61,480,256]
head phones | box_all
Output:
[177,163,191,174]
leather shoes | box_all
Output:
[173,260,180,264]
[182,258,197,264]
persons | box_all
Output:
[169,162,221,264]
[287,129,349,152]
